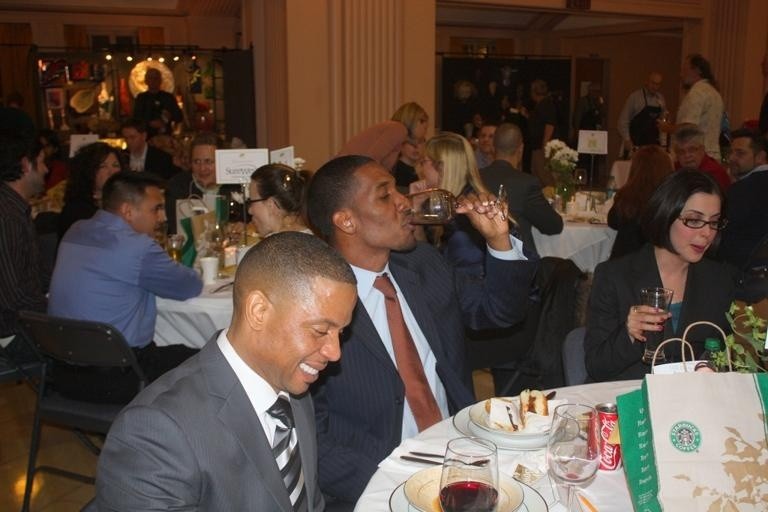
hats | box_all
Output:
[145,69,163,87]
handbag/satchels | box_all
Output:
[615,320,767,511]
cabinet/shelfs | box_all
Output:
[28,41,257,149]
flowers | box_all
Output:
[544,139,579,212]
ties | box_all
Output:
[265,394,311,512]
[373,273,442,433]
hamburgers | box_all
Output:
[519,388,549,425]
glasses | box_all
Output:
[676,214,730,234]
[420,159,433,164]
[245,197,270,207]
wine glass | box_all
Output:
[574,169,587,191]
[229,200,241,242]
[406,184,509,224]
[544,404,601,512]
[438,437,500,512]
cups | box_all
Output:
[566,201,579,221]
[640,286,674,366]
[200,256,219,285]
[167,234,185,263]
[662,110,671,123]
[204,223,223,257]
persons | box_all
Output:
[674,123,731,193]
[0,132,53,364]
[164,132,243,235]
[390,137,426,188]
[36,128,74,200]
[573,81,604,169]
[46,171,204,405]
[607,145,672,260]
[675,54,724,162]
[305,154,541,512]
[134,68,182,172]
[474,123,500,169]
[56,142,122,239]
[409,132,520,254]
[709,129,768,303]
[521,79,560,187]
[479,123,564,260]
[334,102,429,172]
[583,168,744,383]
[95,230,359,512]
[617,70,671,161]
[248,163,314,235]
[0,92,34,129]
[499,79,531,161]
[457,113,483,141]
[120,118,184,178]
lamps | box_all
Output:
[126,56,133,62]
[147,52,153,61]
[158,57,165,63]
[191,52,197,61]
[105,54,112,61]
[173,53,179,62]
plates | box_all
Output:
[389,478,549,512]
[452,398,579,451]
[470,397,568,437]
[404,465,524,512]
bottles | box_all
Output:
[698,337,722,360]
[587,402,624,471]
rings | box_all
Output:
[634,307,637,312]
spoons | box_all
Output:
[409,451,491,467]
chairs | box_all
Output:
[562,327,588,386]
[495,257,585,398]
[0,292,46,395]
[13,310,151,511]
